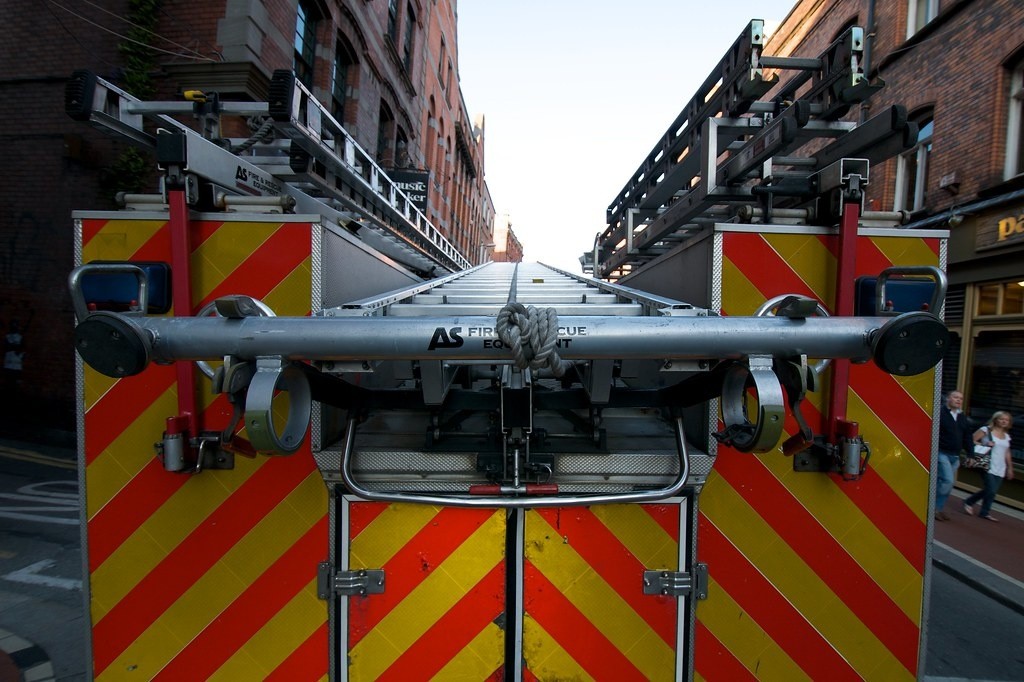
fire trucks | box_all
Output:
[71,18,949,682]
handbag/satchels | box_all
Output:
[964,425,992,472]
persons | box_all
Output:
[963,411,1014,522]
[934,391,973,521]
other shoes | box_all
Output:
[963,498,972,515]
[934,512,942,521]
[979,514,997,522]
[940,511,950,520]
